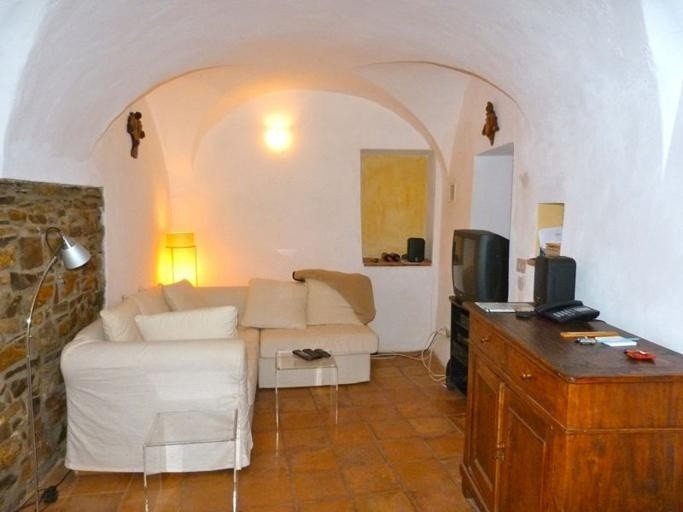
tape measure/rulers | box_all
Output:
[561,332,620,338]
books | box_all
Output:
[474,302,536,312]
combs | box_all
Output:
[560,329,618,336]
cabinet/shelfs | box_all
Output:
[458,302,682,512]
[445,294,470,397]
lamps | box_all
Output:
[17,225,93,512]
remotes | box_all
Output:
[293,349,311,361]
[315,348,332,359]
[303,347,317,357]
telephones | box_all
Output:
[535,300,600,324]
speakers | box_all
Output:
[407,237,425,263]
[533,256,577,308]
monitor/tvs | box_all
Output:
[452,228,509,303]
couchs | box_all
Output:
[56,283,380,476]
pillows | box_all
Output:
[99,275,376,342]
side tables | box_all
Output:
[142,407,241,512]
